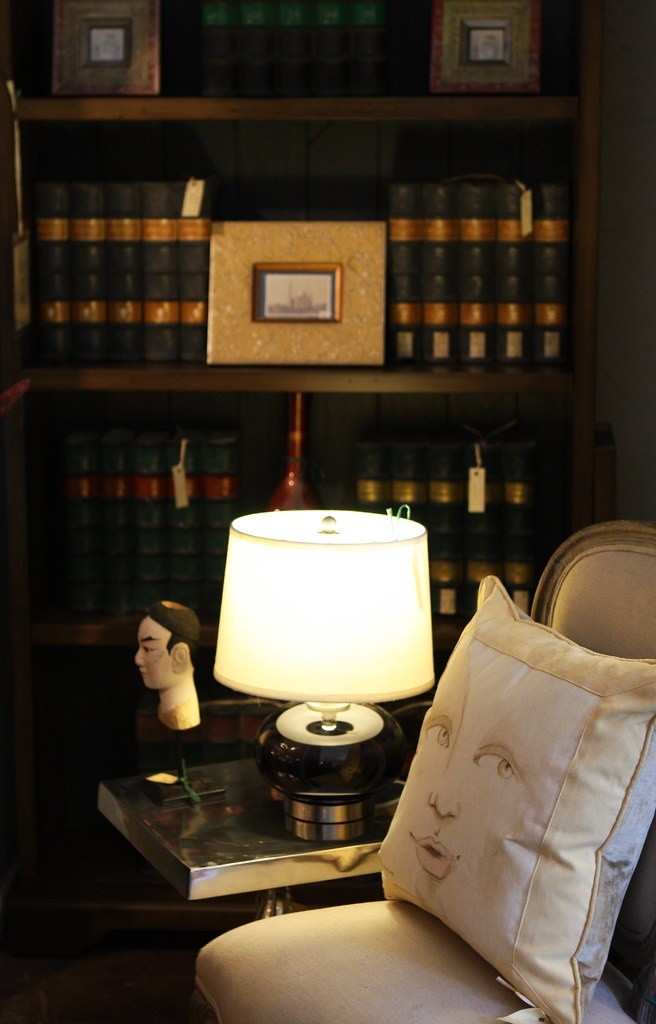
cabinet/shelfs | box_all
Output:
[0,0,598,914]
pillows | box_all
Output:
[379,576,656,948]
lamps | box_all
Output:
[213,510,436,842]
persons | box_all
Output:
[132,599,204,730]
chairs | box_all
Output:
[193,519,656,1024]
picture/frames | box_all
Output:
[208,221,387,367]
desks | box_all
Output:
[97,744,443,919]
